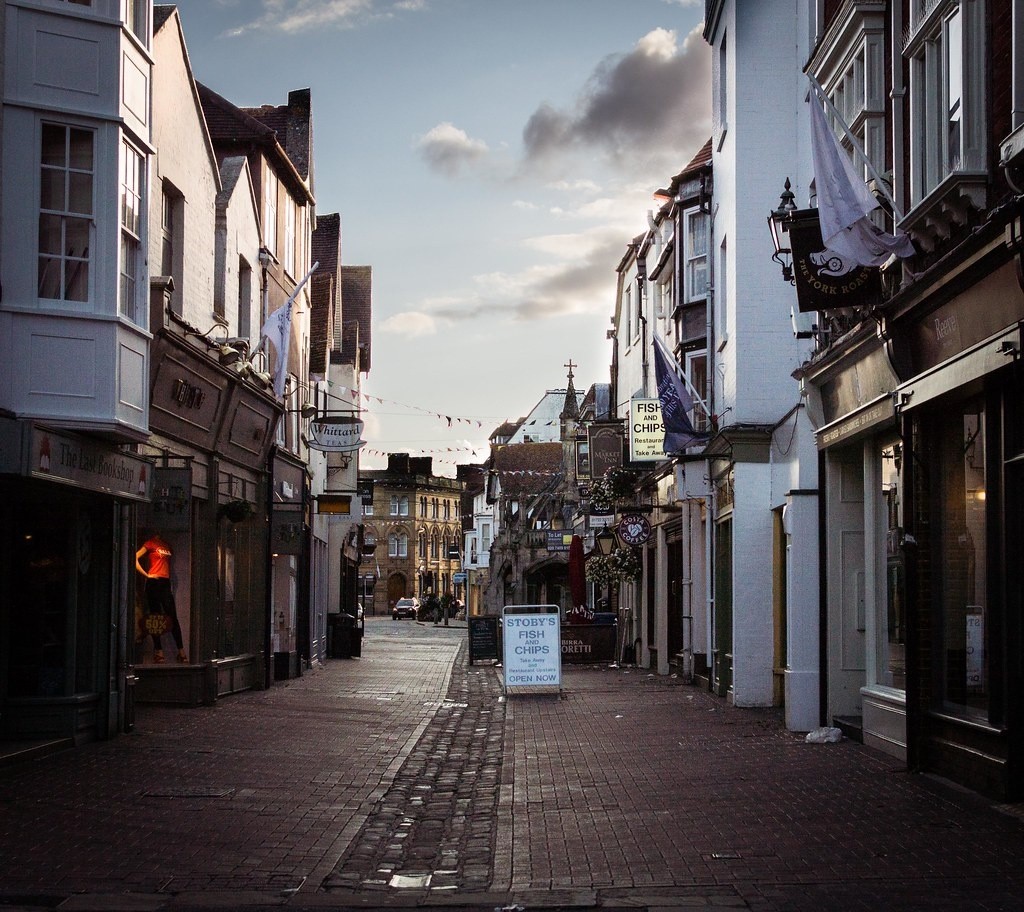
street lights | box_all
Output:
[596,519,616,614]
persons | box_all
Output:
[135,535,188,662]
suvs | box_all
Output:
[392,597,420,620]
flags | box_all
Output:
[260,300,293,402]
[653,339,715,452]
[809,86,918,266]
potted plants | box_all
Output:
[441,590,454,605]
[164,486,188,512]
[221,499,256,523]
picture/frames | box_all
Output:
[575,440,591,480]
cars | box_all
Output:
[358,603,366,620]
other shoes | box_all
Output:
[180,654,189,664]
[154,654,165,664]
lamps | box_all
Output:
[184,323,240,365]
[595,520,615,556]
[765,176,797,286]
[283,385,316,420]
[207,338,253,380]
[966,425,984,469]
[249,352,271,390]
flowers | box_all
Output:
[604,545,642,585]
[601,466,639,501]
[588,479,611,515]
[583,555,609,590]
